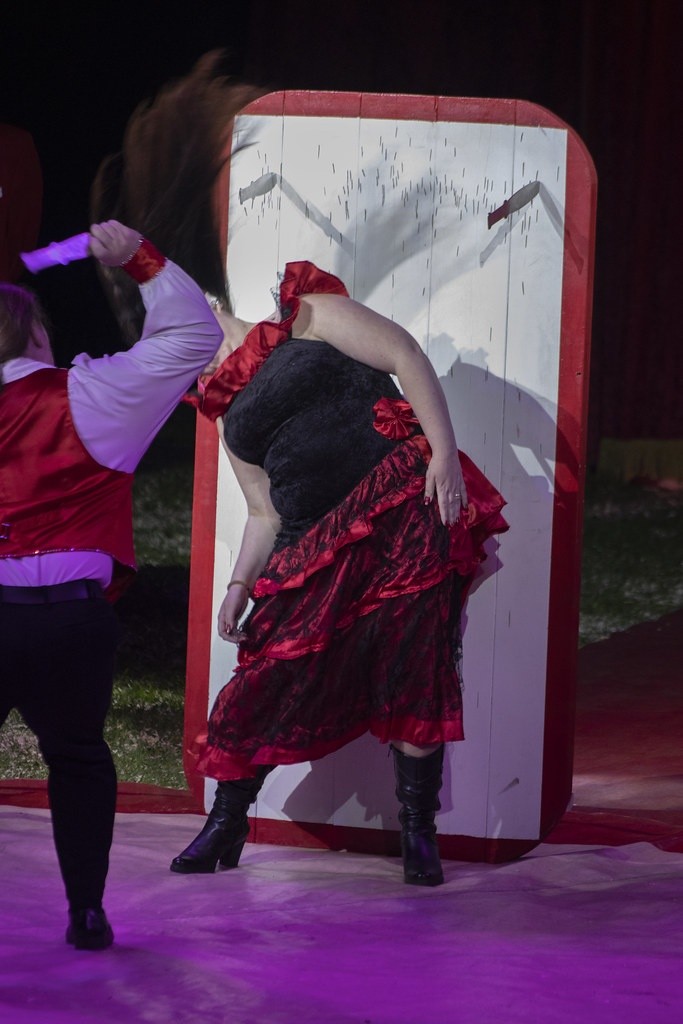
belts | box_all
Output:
[0,578,91,604]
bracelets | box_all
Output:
[227,580,251,596]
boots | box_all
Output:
[387,743,445,885]
[171,765,265,873]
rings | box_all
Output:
[455,494,460,496]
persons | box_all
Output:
[92,87,508,888]
[0,221,224,951]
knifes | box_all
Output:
[19,231,105,273]
[487,182,539,227]
[238,172,277,202]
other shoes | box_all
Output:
[64,905,114,949]
